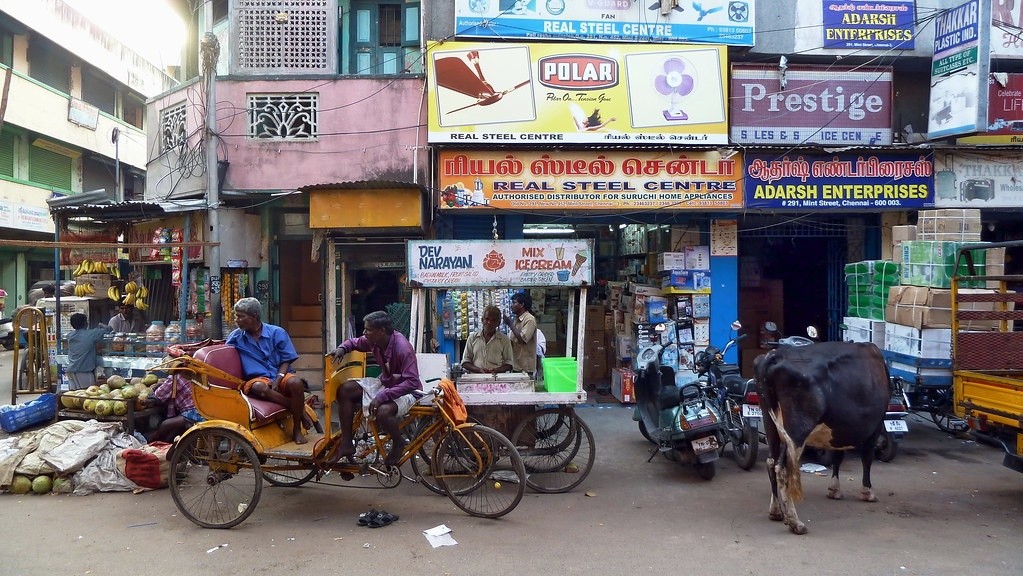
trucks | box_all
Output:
[950,238,1022,474]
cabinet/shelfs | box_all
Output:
[644,222,701,279]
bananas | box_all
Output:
[73,260,120,279]
[74,283,96,297]
[108,281,150,310]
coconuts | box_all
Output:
[61,374,160,416]
[8,474,65,495]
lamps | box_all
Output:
[523,220,577,235]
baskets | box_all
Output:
[0,394,56,433]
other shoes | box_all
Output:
[583,384,595,391]
[596,389,608,395]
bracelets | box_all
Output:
[277,372,285,377]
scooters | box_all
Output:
[631,322,729,481]
[762,320,912,467]
[0,318,16,350]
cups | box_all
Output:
[556,247,564,260]
[557,270,570,281]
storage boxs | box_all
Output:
[76,276,125,298]
[842,209,1017,386]
[540,245,713,404]
[736,256,785,380]
[0,393,60,433]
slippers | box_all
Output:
[357,509,379,526]
[368,510,399,528]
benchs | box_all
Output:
[190,345,310,452]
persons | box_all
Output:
[460,306,514,375]
[32,281,56,347]
[503,293,546,376]
[65,313,113,390]
[225,297,308,445]
[154,374,204,441]
[107,296,146,333]
[325,311,425,466]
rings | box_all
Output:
[373,406,377,411]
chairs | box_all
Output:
[11,306,56,406]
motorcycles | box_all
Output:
[690,321,769,472]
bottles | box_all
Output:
[62,319,205,357]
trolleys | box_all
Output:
[402,373,596,496]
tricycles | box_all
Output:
[890,374,970,436]
[149,335,526,530]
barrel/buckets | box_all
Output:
[547,361,577,391]
[542,357,576,390]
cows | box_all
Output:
[754,341,892,535]
